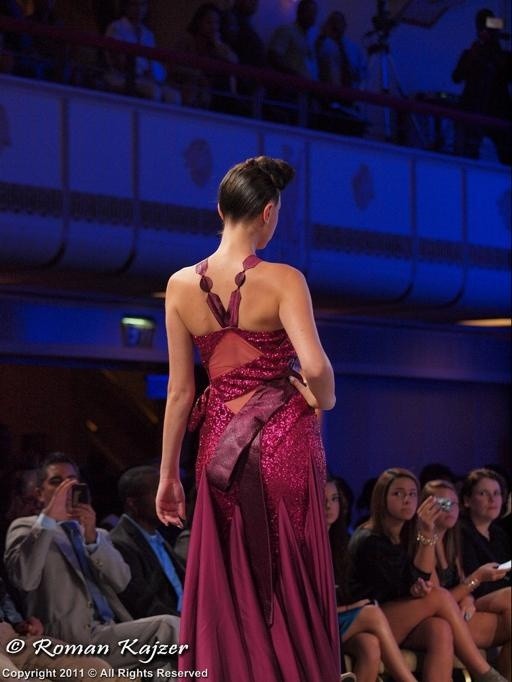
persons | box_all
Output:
[0,444,197,682]
[324,463,512,682]
[156,156,337,682]
[452,8,512,168]
[0,0,364,137]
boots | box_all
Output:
[474,665,510,682]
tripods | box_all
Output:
[364,52,432,151]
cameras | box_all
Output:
[67,483,91,513]
[431,496,452,513]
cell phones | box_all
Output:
[496,560,512,571]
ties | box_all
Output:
[59,520,116,626]
